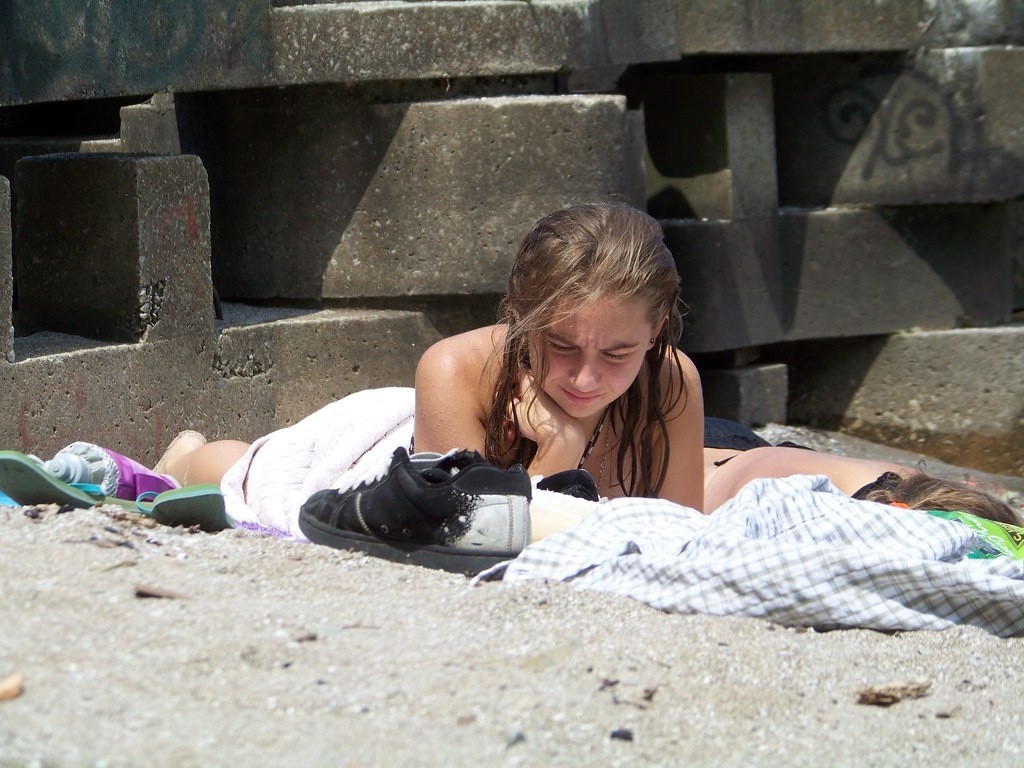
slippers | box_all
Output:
[1,450,152,515]
[137,482,226,533]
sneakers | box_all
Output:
[530,468,599,503]
[298,446,533,581]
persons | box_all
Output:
[702,418,1017,524]
[151,203,704,527]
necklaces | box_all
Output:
[597,423,612,492]
